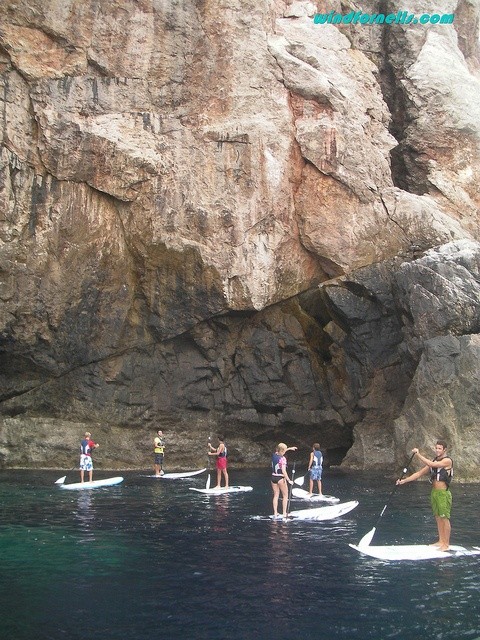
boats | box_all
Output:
[189,484,254,495]
[139,466,207,480]
[249,500,361,522]
[347,541,480,562]
[291,487,341,506]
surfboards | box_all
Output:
[291,487,340,503]
[188,486,254,496]
[145,468,206,480]
[261,500,359,521]
[349,543,480,560]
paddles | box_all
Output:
[294,469,310,487]
[359,451,418,547]
[205,445,210,488]
[55,447,96,484]
[159,446,165,475]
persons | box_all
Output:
[207,434,229,489]
[396,441,454,552]
[80,432,99,484]
[270,443,298,517]
[306,443,323,497]
[154,429,165,476]
